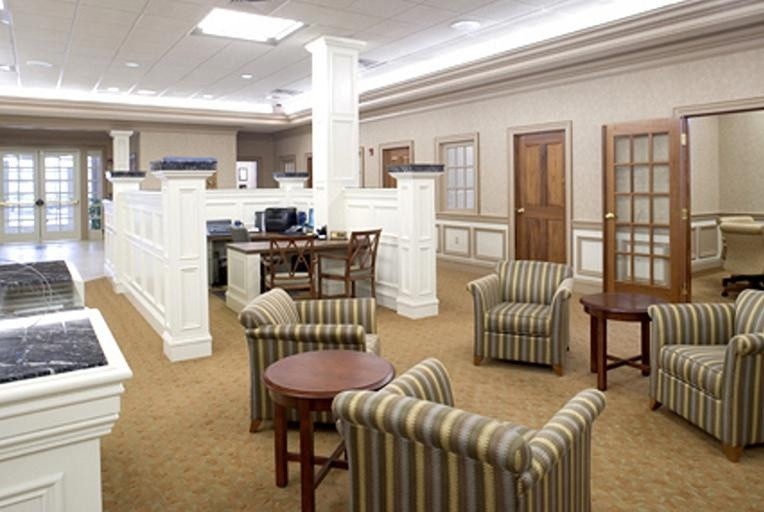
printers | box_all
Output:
[266,208,298,231]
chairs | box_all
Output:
[265,234,317,301]
[239,287,378,432]
[716,216,764,296]
[647,289,764,463]
[317,228,382,299]
[331,358,607,512]
[466,259,575,375]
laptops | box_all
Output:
[207,220,231,237]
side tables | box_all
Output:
[580,293,662,391]
[263,350,396,510]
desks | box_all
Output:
[207,226,314,292]
[225,239,370,316]
